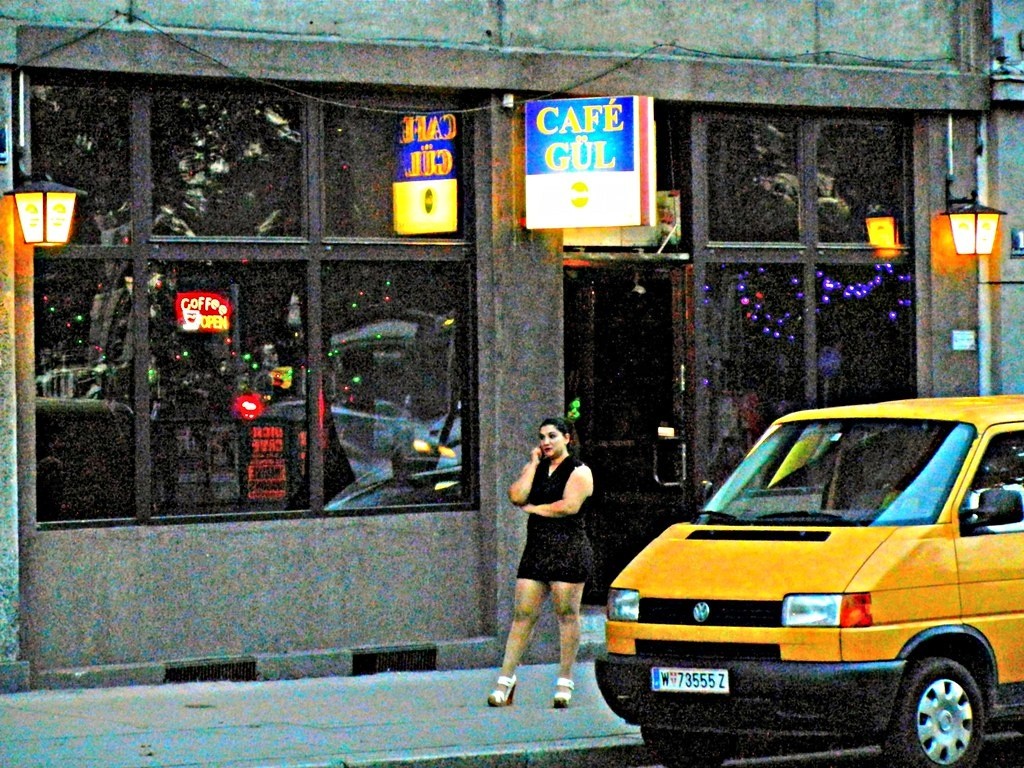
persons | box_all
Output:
[488,416,594,708]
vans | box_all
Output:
[591,394,1024,768]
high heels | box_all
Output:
[553,678,574,707]
[488,674,517,706]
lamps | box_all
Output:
[941,173,1008,257]
[2,147,88,245]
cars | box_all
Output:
[35,315,464,508]
